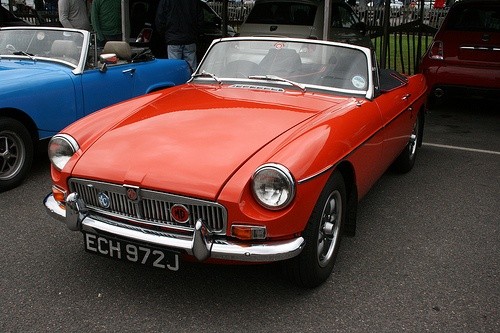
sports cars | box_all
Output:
[0,27,194,191]
[42,36,431,287]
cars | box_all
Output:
[417,0,500,108]
[412,0,446,19]
[91,0,239,59]
[386,0,403,17]
[237,0,377,64]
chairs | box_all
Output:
[50,40,78,67]
[98,41,131,68]
[328,53,367,81]
[259,48,300,74]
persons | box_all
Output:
[347,0,455,19]
[9,0,89,31]
[156,0,200,73]
[91,0,122,47]
